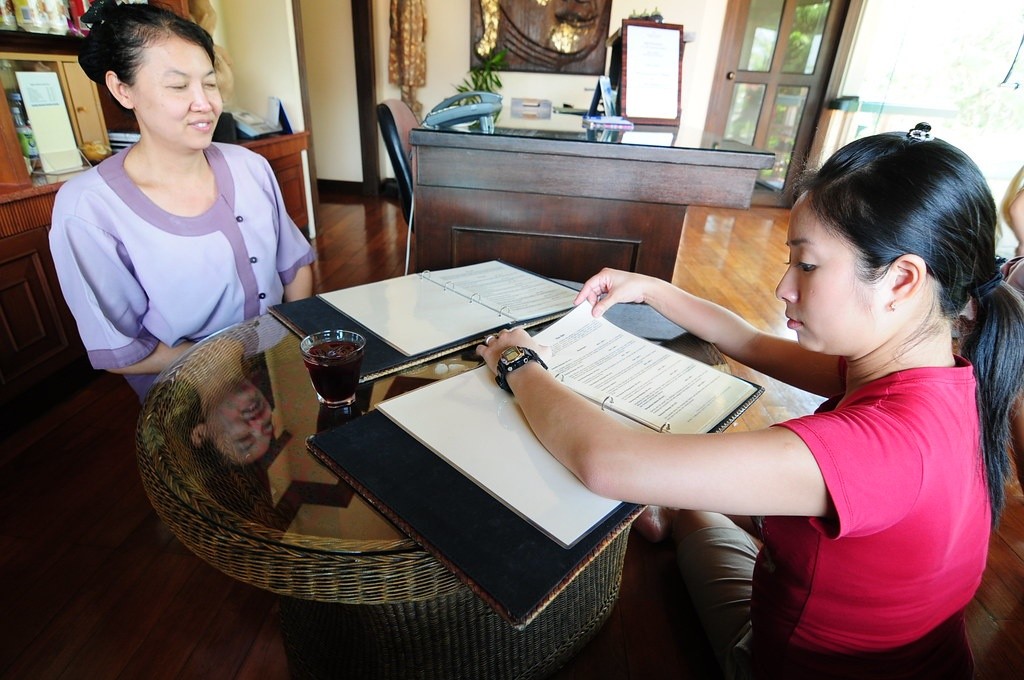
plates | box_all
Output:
[108,132,141,154]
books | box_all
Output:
[302,301,768,626]
[262,259,588,387]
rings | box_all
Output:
[485,335,495,346]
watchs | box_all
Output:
[495,346,549,396]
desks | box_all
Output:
[133,265,735,680]
[407,114,775,294]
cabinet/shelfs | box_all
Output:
[0,226,94,438]
[268,152,310,238]
[1,1,308,236]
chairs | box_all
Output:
[375,103,421,233]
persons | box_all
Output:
[47,0,318,415]
[475,123,1024,680]
[993,181,1024,503]
[174,317,290,471]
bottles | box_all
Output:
[8,93,40,158]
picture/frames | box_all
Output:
[621,18,683,128]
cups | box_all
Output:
[300,330,366,408]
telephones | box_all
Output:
[424,90,503,131]
